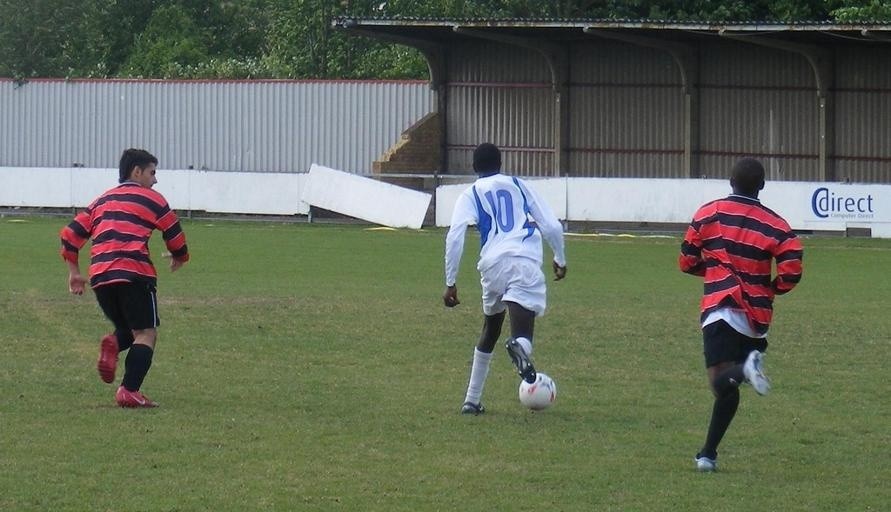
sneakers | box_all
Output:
[695,452,714,471]
[504,337,536,384]
[461,401,485,416]
[98,334,118,384]
[116,385,159,407]
[743,350,770,397]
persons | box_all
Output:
[443,143,567,413]
[679,159,803,472]
[60,149,189,408]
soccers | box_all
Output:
[518,373,556,409]
